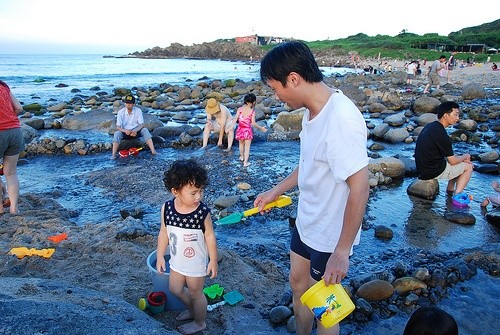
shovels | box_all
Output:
[7,247,55,259]
[205,290,244,312]
[214,194,291,226]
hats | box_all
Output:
[122,95,135,105]
[205,99,220,115]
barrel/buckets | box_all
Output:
[299,278,355,330]
[147,245,191,310]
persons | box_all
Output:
[415,101,474,200]
[225,93,267,166]
[253,40,370,335]
[481,196,500,206]
[492,63,497,71]
[352,50,476,95]
[113,95,154,154]
[487,55,491,64]
[203,98,236,148]
[156,160,217,334]
[0,80,25,216]
